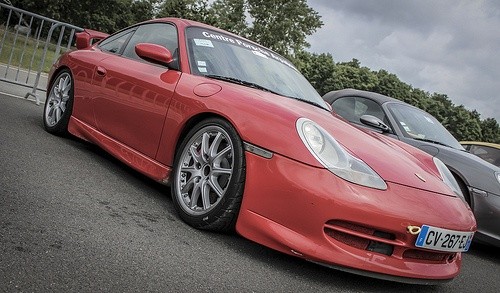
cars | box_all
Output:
[458,141,500,169]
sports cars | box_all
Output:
[42,17,477,285]
[320,88,500,249]
